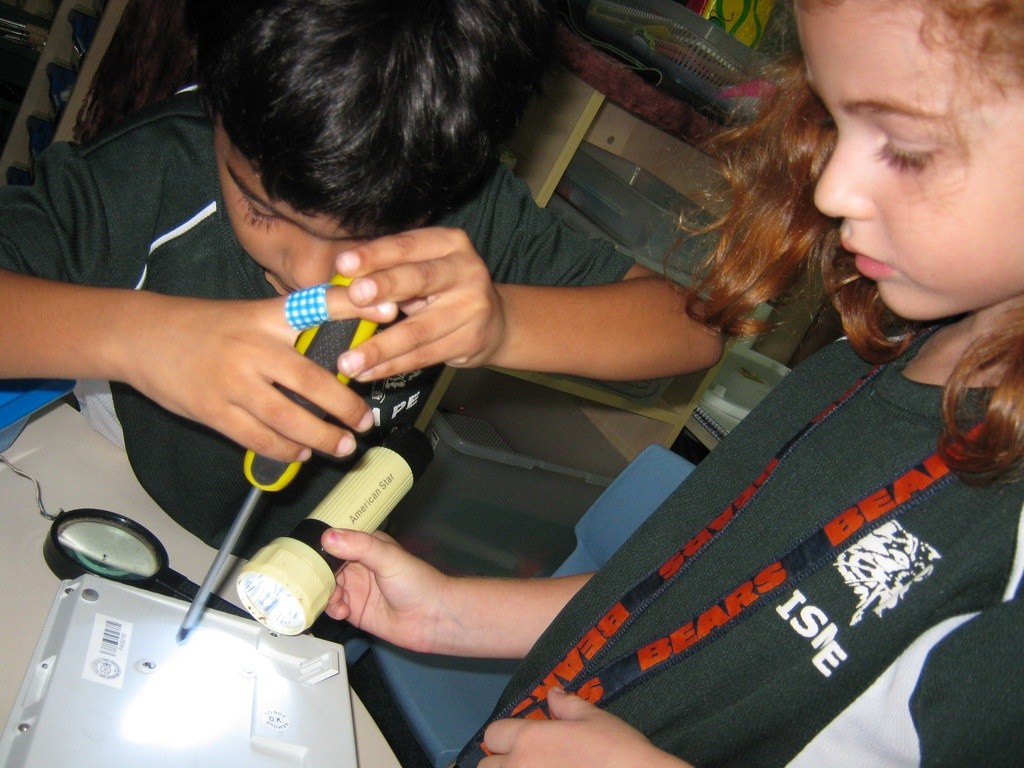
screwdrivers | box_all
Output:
[174,270,383,646]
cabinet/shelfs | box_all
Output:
[413,64,762,488]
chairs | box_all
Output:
[336,443,696,768]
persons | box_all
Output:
[0,0,724,559]
[319,0,1024,768]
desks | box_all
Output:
[0,398,401,768]
